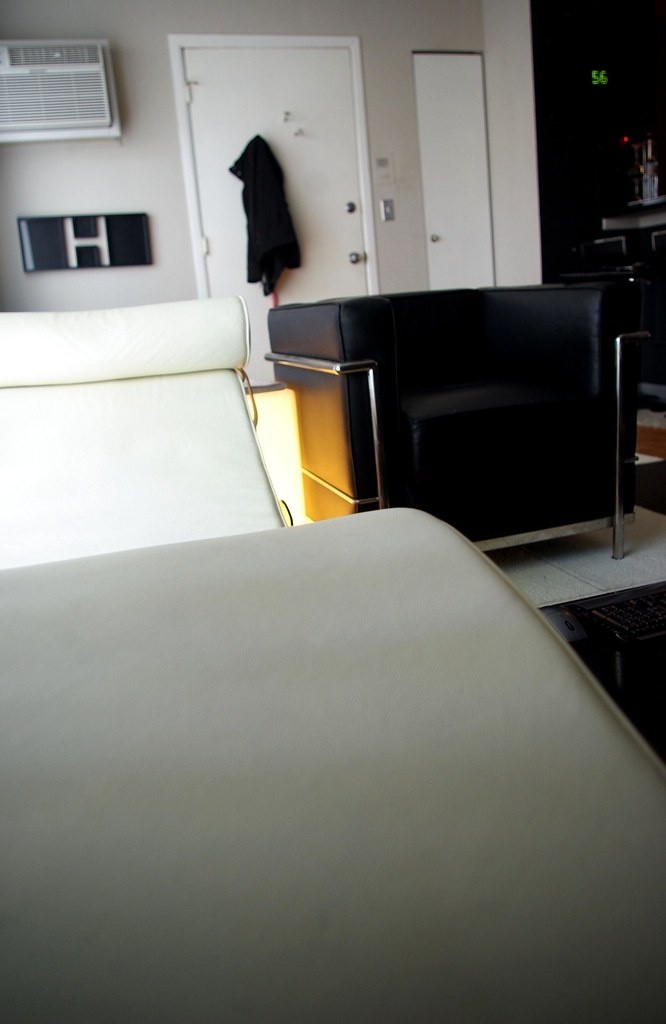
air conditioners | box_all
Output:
[0,40,123,143]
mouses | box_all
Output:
[539,607,587,642]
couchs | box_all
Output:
[0,506,666,1024]
[265,281,652,558]
[0,296,283,573]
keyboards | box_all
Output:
[593,591,666,640]
